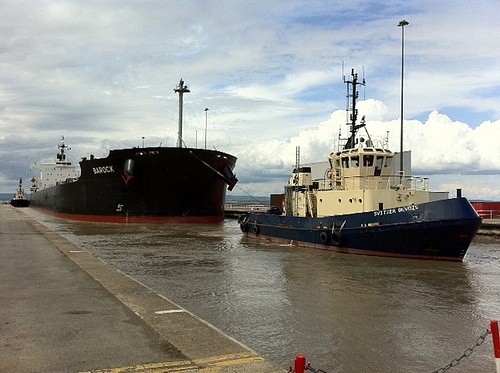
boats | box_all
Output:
[240,17,484,265]
[11,177,31,208]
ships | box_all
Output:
[31,78,241,227]
